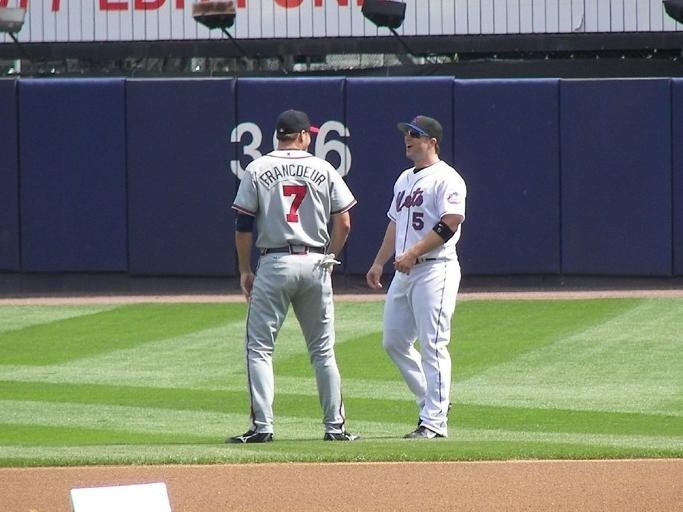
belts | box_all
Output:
[262,243,327,255]
[414,256,438,266]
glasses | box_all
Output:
[402,130,426,139]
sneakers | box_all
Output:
[225,429,275,443]
[403,399,452,439]
[323,426,361,440]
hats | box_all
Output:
[276,108,320,135]
[397,114,443,144]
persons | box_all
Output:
[364,115,466,440]
[225,109,359,444]
